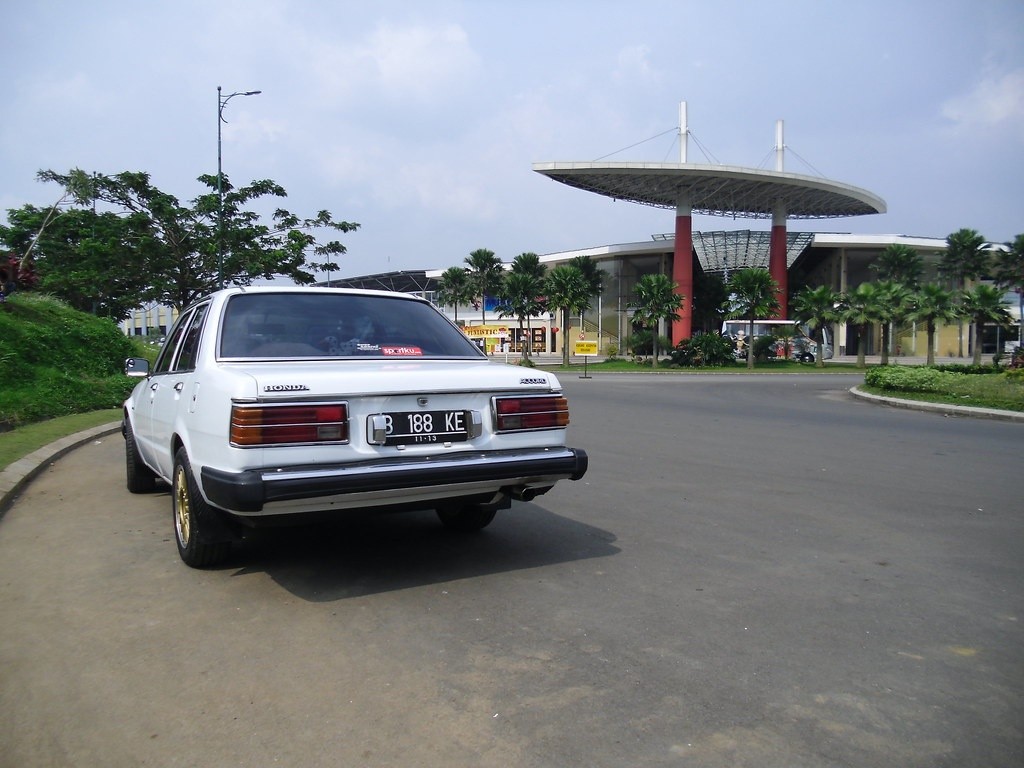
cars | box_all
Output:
[120,285,589,572]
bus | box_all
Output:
[722,320,834,363]
[970,322,1021,354]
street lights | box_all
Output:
[216,85,261,287]
[959,243,992,358]
